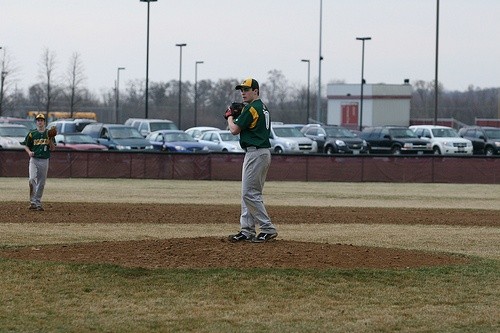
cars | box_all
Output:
[54,133,107,150]
[195,131,245,152]
[146,129,208,151]
[0,117,36,151]
[268,120,318,154]
[80,122,154,151]
[179,126,220,141]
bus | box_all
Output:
[28,111,96,125]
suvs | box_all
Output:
[301,120,500,157]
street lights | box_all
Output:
[139,0,158,119]
[301,59,310,124]
[194,61,204,127]
[355,37,372,132]
[115,66,125,124]
[176,43,187,130]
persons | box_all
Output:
[224,78,278,243]
[23,114,56,211]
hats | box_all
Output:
[36,114,45,120]
[235,78,259,90]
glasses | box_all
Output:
[241,88,251,92]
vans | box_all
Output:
[126,118,175,138]
[46,118,97,134]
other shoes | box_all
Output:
[37,202,41,207]
[30,203,37,209]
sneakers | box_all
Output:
[232,231,255,241]
[252,232,278,243]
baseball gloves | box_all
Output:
[223,102,249,120]
[48,125,58,138]
